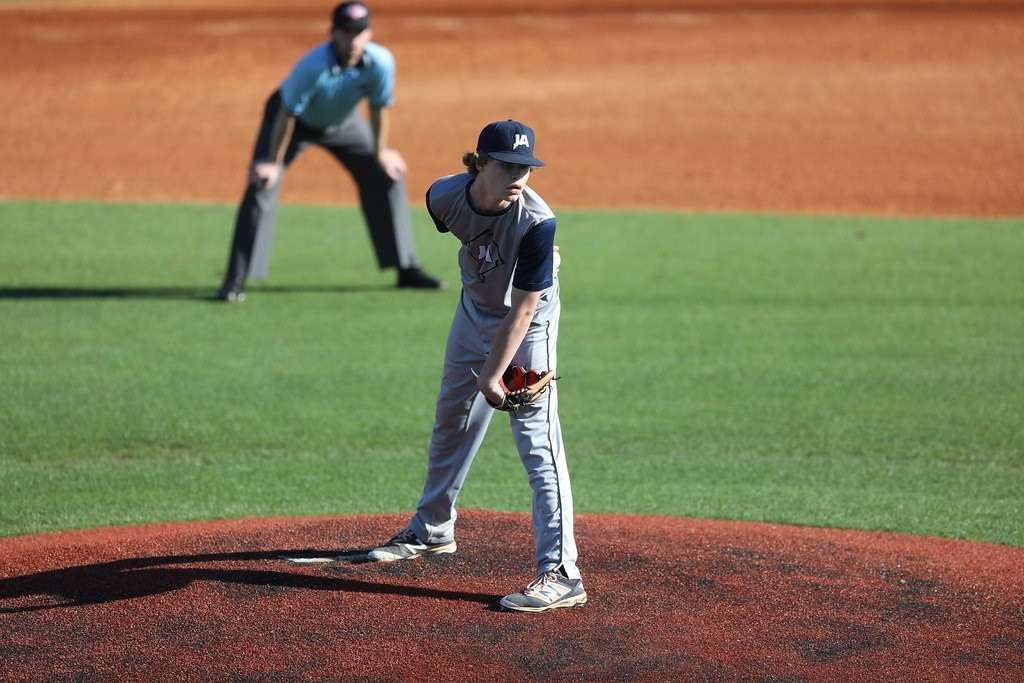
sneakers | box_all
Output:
[499,570,587,612]
[366,527,457,562]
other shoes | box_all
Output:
[398,268,442,289]
[218,276,244,301]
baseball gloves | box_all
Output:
[485,361,558,411]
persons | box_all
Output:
[217,0,447,304]
[367,119,588,613]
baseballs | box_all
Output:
[552,251,563,267]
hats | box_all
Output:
[476,119,544,166]
[333,2,369,32]
